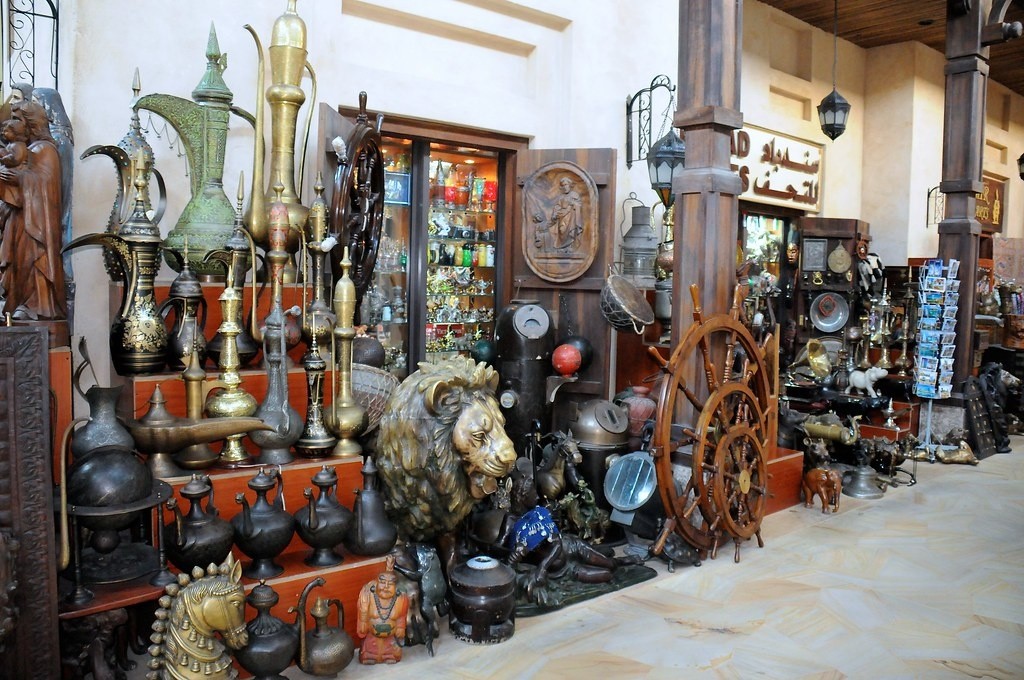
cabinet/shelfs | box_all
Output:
[616,290,805,518]
[109,278,393,680]
[860,345,921,442]
[315,102,618,432]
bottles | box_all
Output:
[434,158,455,210]
[427,240,496,267]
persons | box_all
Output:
[0,81,73,323]
[532,211,556,254]
[551,177,584,254]
[357,569,409,665]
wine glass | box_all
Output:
[475,177,497,213]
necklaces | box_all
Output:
[373,593,398,620]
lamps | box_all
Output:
[1017,153,1024,181]
[816,1,851,140]
[627,74,687,210]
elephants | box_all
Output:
[802,468,842,514]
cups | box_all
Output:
[457,185,469,211]
[383,307,391,320]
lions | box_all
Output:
[375,355,517,540]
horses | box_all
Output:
[512,429,584,518]
[146,552,248,680]
